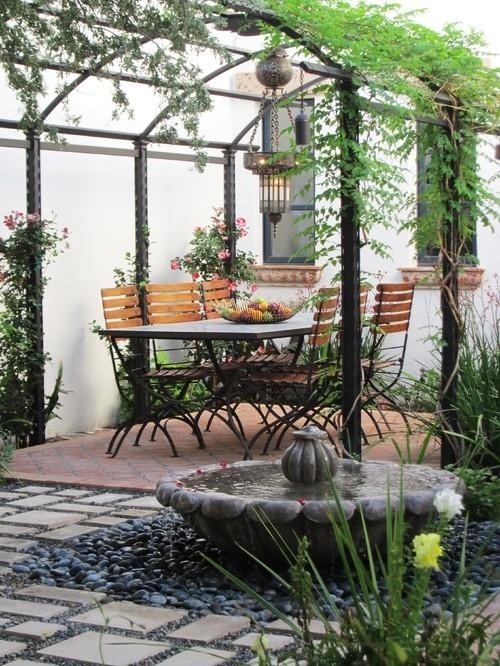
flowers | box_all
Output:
[171,207,258,282]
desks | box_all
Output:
[98,315,376,446]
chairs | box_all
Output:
[323,278,415,442]
[202,279,235,320]
[273,283,383,449]
[142,282,247,443]
[100,285,206,460]
[239,285,341,459]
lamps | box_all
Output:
[244,44,313,241]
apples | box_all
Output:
[223,297,292,321]
